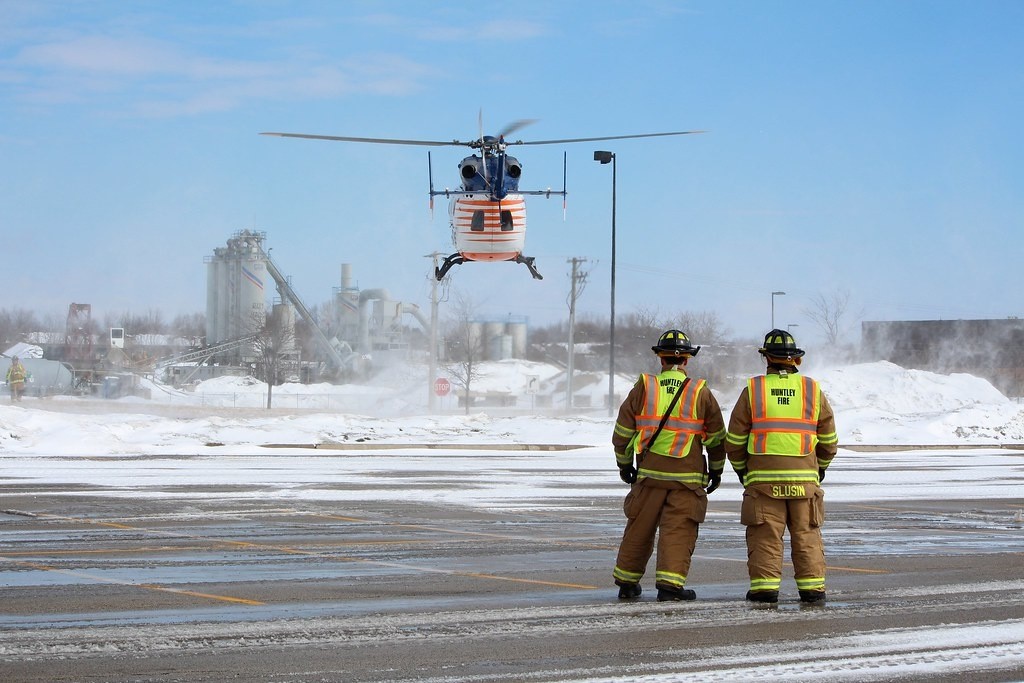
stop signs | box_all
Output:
[433,377,451,396]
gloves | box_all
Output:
[707,473,721,494]
[819,470,825,483]
[620,466,636,484]
[739,475,746,489]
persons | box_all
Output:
[5,355,27,403]
[723,330,837,603]
[612,330,727,600]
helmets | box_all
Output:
[758,329,805,359]
[651,330,702,357]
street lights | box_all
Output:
[592,148,619,416]
[770,289,787,334]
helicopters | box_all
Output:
[257,118,714,283]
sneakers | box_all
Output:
[746,589,779,603]
[799,592,826,603]
[618,584,641,598]
[657,590,696,602]
